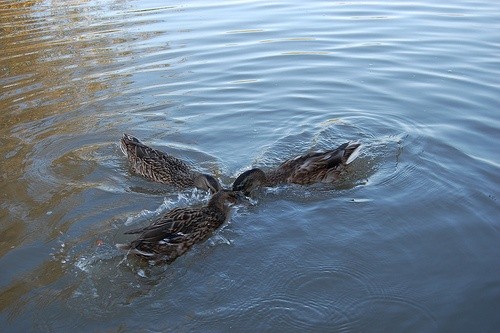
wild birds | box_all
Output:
[121,190,237,266]
[119,132,221,193]
[231,140,361,194]
